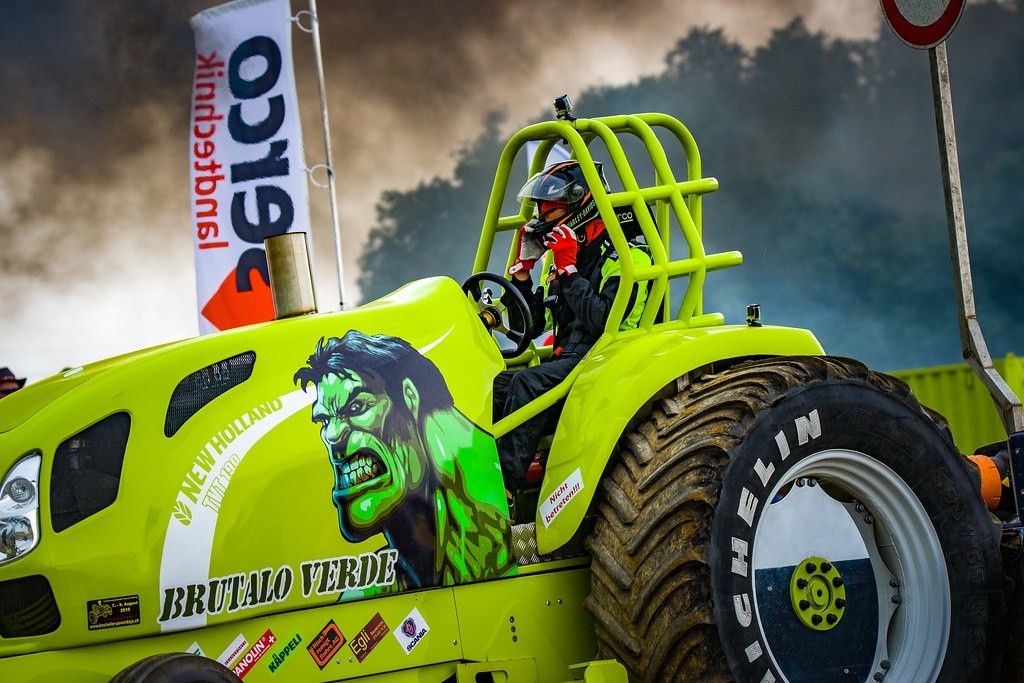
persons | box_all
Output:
[0,366,25,399]
[492,159,666,510]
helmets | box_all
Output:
[525,160,609,241]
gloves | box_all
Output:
[543,223,578,282]
[508,216,550,275]
